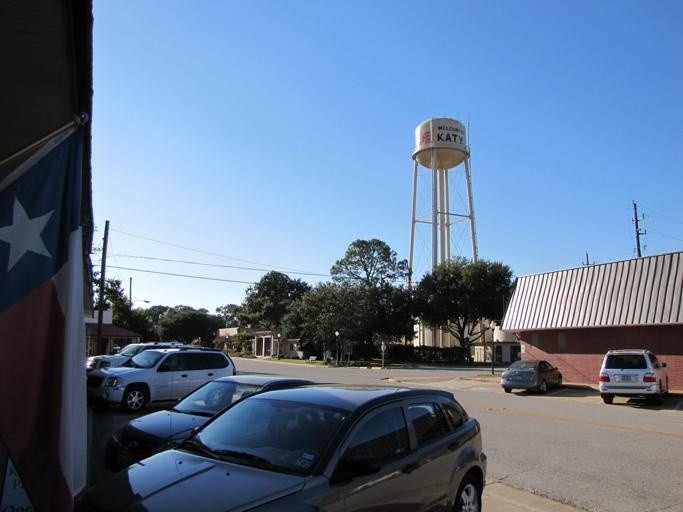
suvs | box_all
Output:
[84,341,184,371]
[86,347,235,412]
[599,348,668,405]
[73,386,485,512]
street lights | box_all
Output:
[334,330,339,367]
[225,335,228,357]
[489,320,496,374]
[276,333,280,358]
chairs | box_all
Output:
[281,410,321,449]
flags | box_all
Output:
[0,111,88,512]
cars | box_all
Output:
[501,360,561,393]
[103,374,314,472]
